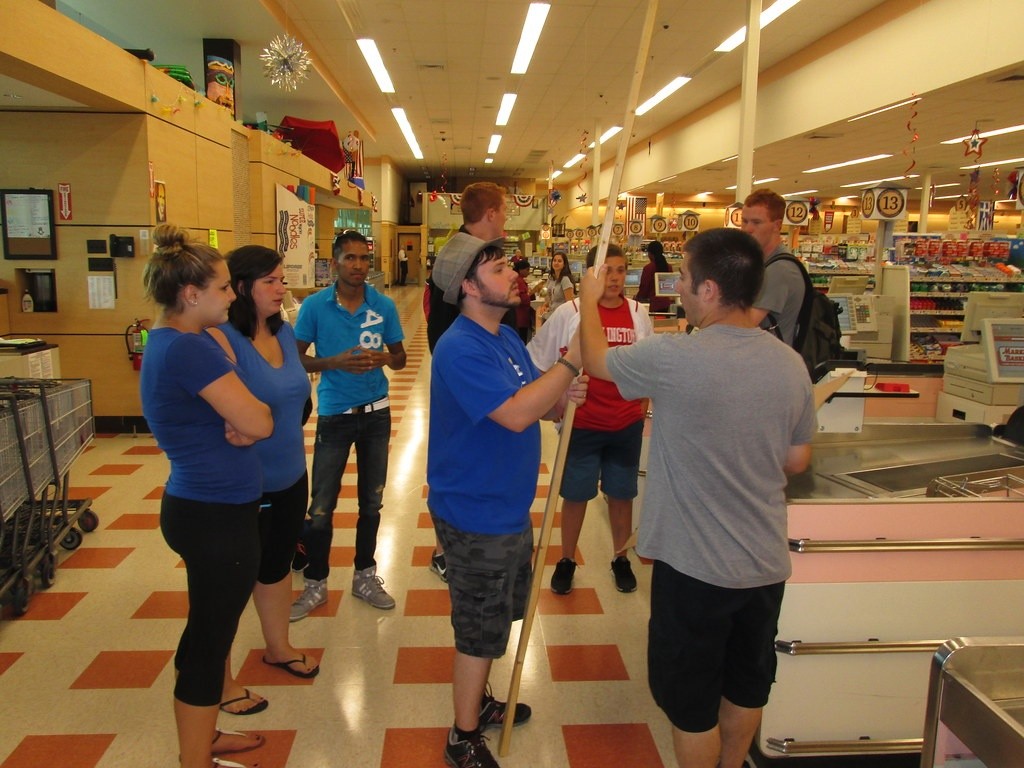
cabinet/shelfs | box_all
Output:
[566,230,1024,365]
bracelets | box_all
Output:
[558,359,579,376]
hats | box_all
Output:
[431,232,503,306]
[514,258,530,271]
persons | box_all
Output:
[220,246,321,716]
[528,244,655,593]
[399,245,408,286]
[291,230,406,620]
[427,233,590,768]
[581,229,818,768]
[140,223,273,768]
[511,250,525,262]
[636,242,672,310]
[542,251,574,323]
[740,188,806,346]
[512,261,535,343]
[424,183,517,581]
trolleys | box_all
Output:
[0,374,98,617]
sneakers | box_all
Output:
[429,549,448,583]
[551,558,576,595]
[478,680,531,726]
[351,566,395,610]
[292,519,311,572]
[611,555,636,592]
[443,724,501,768]
[289,577,327,622]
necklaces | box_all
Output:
[336,292,341,305]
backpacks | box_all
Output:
[764,252,843,385]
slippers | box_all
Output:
[212,728,263,755]
[219,687,267,715]
[263,650,319,678]
[212,757,246,768]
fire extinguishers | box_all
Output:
[125,317,151,371]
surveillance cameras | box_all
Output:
[600,95,604,97]
[663,25,669,29]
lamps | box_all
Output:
[831,201,836,209]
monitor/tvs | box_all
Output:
[624,269,643,288]
[654,273,681,296]
[828,276,869,294]
[981,318,1024,384]
[961,291,1024,344]
[534,256,553,268]
[568,261,581,274]
[824,293,857,334]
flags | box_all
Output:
[626,196,647,237]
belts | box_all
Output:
[343,396,390,414]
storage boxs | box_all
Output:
[917,336,942,355]
[933,335,963,355]
